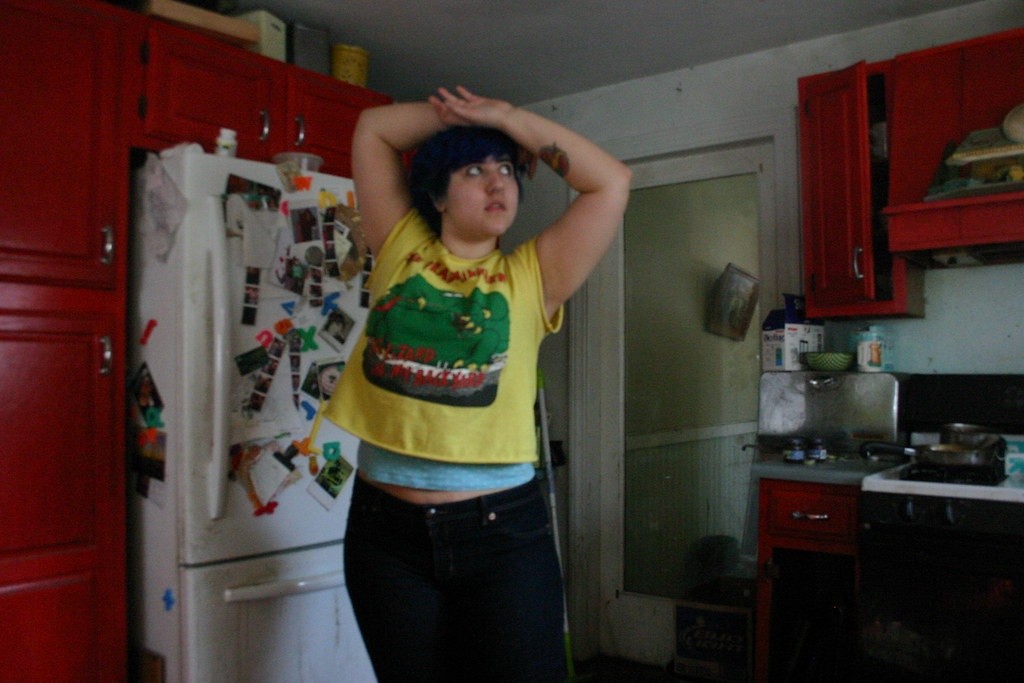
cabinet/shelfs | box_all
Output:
[130,12,392,179]
[0,1,139,683]
[798,58,924,320]
[755,476,902,683]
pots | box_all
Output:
[858,440,989,469]
[939,422,992,450]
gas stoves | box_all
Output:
[860,430,1024,535]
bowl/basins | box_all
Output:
[271,152,323,172]
[798,351,856,373]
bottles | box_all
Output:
[213,126,238,159]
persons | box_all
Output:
[322,87,632,683]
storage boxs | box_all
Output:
[141,0,260,50]
[236,10,287,63]
[761,294,825,371]
[288,19,331,75]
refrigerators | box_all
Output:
[125,151,378,683]
